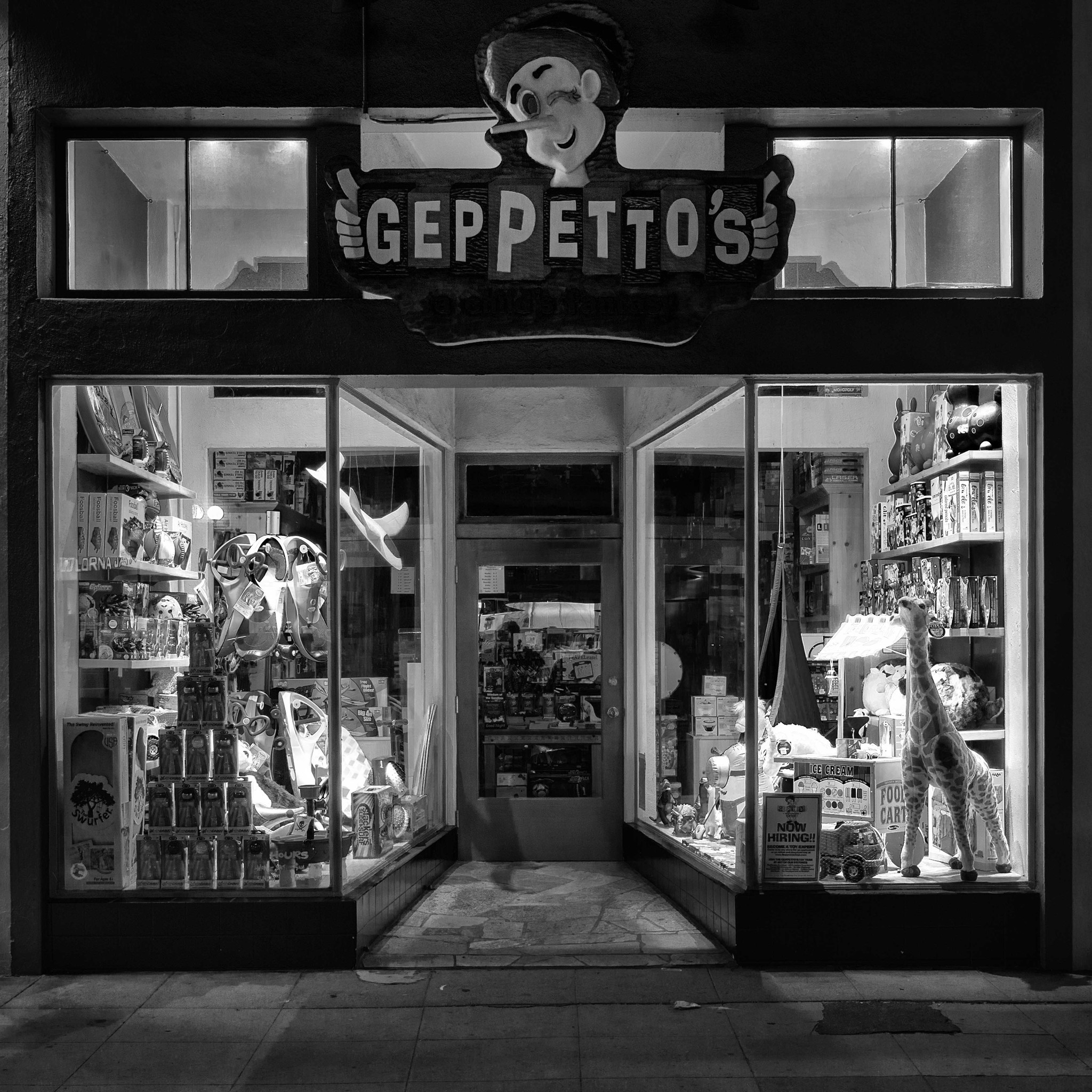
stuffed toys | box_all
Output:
[648,596,1013,881]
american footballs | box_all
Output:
[167,532,185,566]
[144,528,175,561]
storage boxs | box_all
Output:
[89,491,106,558]
[320,707,392,739]
[792,754,907,833]
[272,676,390,708]
[689,673,743,737]
[61,707,149,890]
[927,764,1008,871]
[107,493,146,562]
[213,449,327,524]
[76,491,91,561]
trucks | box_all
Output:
[818,818,883,882]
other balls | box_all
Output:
[558,703,578,722]
[392,804,409,840]
[368,761,405,797]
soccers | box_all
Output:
[532,783,549,797]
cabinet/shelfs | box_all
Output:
[75,453,191,671]
[870,447,1007,745]
[785,482,862,634]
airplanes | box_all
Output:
[302,452,411,574]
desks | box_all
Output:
[481,730,603,800]
